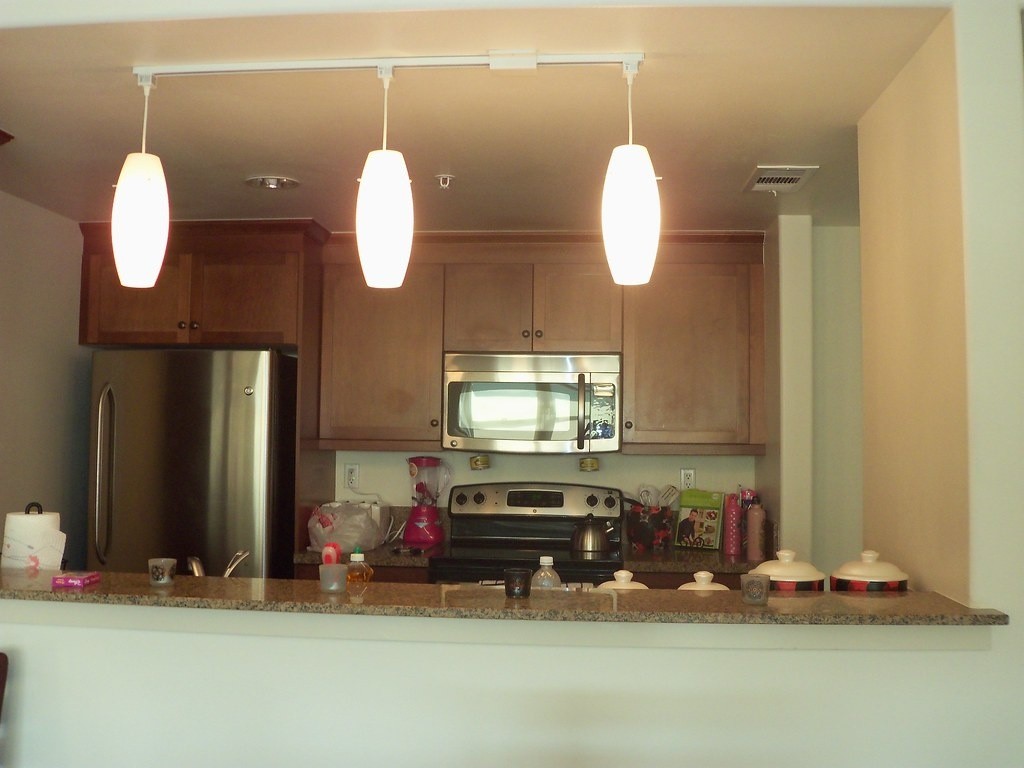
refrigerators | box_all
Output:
[87,349,296,578]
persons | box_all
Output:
[678,509,698,544]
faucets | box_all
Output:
[186,556,205,576]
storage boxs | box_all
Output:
[52,572,101,587]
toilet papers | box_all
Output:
[0,510,66,571]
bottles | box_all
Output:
[597,570,648,589]
[678,570,730,591]
[829,549,909,592]
[347,545,374,583]
[724,495,742,556]
[626,503,672,549]
[748,495,765,561]
[748,549,825,591]
[532,556,561,588]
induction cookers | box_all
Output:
[428,481,625,573]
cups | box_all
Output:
[740,573,771,605]
[504,567,531,598]
[319,564,348,592]
[147,558,177,587]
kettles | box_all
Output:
[570,513,614,551]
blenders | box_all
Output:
[403,455,451,550]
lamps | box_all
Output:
[356,66,414,289]
[600,61,662,287]
[111,75,169,290]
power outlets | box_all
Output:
[343,463,358,489]
[680,468,696,489]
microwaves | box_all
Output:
[440,352,622,453]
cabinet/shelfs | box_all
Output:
[79,223,772,455]
[294,563,430,584]
[631,572,744,592]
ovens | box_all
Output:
[319,500,390,544]
